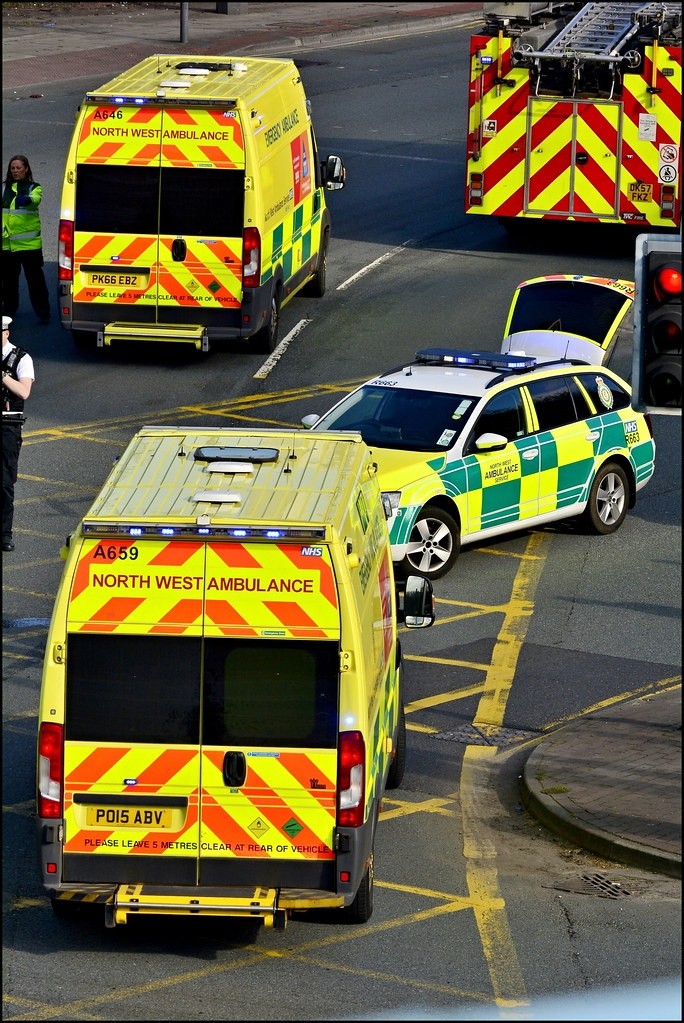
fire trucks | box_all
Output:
[465,3,683,229]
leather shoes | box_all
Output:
[2,534,14,550]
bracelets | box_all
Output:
[2,372,6,381]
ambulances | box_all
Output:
[35,425,438,930]
[56,52,349,356]
[300,271,658,581]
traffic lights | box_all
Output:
[630,230,683,418]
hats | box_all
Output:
[2,315,13,332]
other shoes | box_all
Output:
[4,305,19,317]
[37,309,51,320]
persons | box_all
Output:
[2,316,35,551]
[1,155,51,325]
